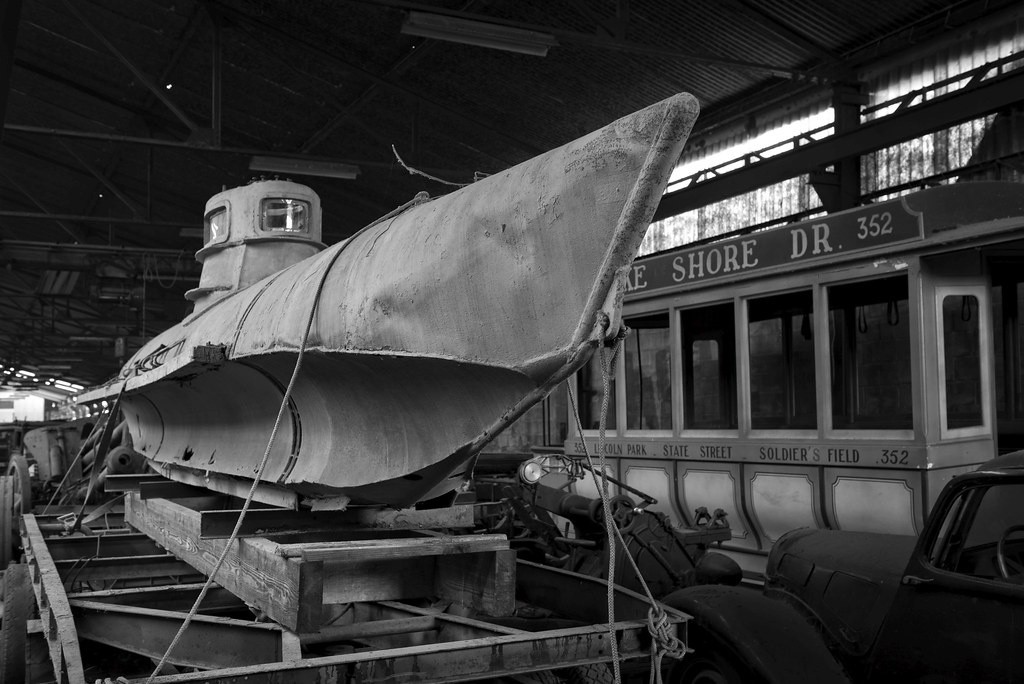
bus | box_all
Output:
[531,153,1024,590]
